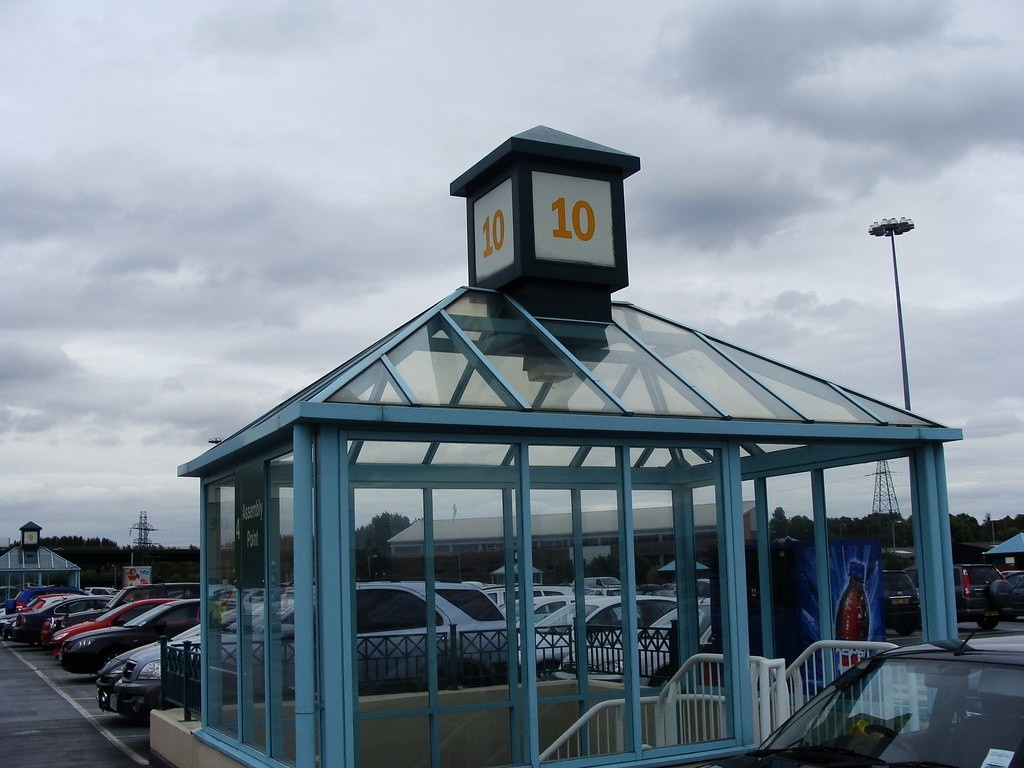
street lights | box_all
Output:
[112,565,116,588]
[866,214,915,411]
[368,556,377,579]
[893,521,902,554]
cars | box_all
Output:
[169,584,294,629]
[95,600,278,709]
[1000,571,1024,620]
[0,583,47,603]
[481,578,710,685]
[0,592,201,674]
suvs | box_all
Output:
[50,583,201,637]
[882,569,920,637]
[903,564,1014,630]
[5,587,93,615]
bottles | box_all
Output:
[834,561,870,676]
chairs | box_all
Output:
[936,668,1023,768]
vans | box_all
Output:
[115,580,508,724]
[573,577,621,595]
